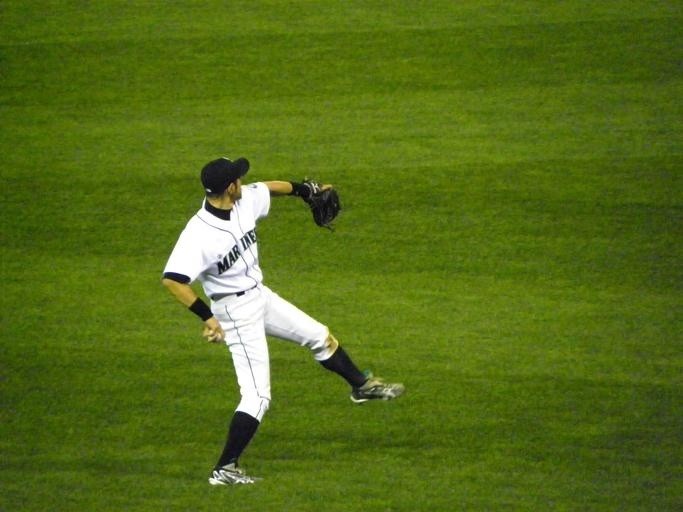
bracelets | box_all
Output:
[188,297,214,321]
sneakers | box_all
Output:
[350,378,405,403]
[207,465,255,485]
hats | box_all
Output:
[200,157,250,194]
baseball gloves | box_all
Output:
[302,179,339,231]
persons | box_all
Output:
[159,155,409,488]
